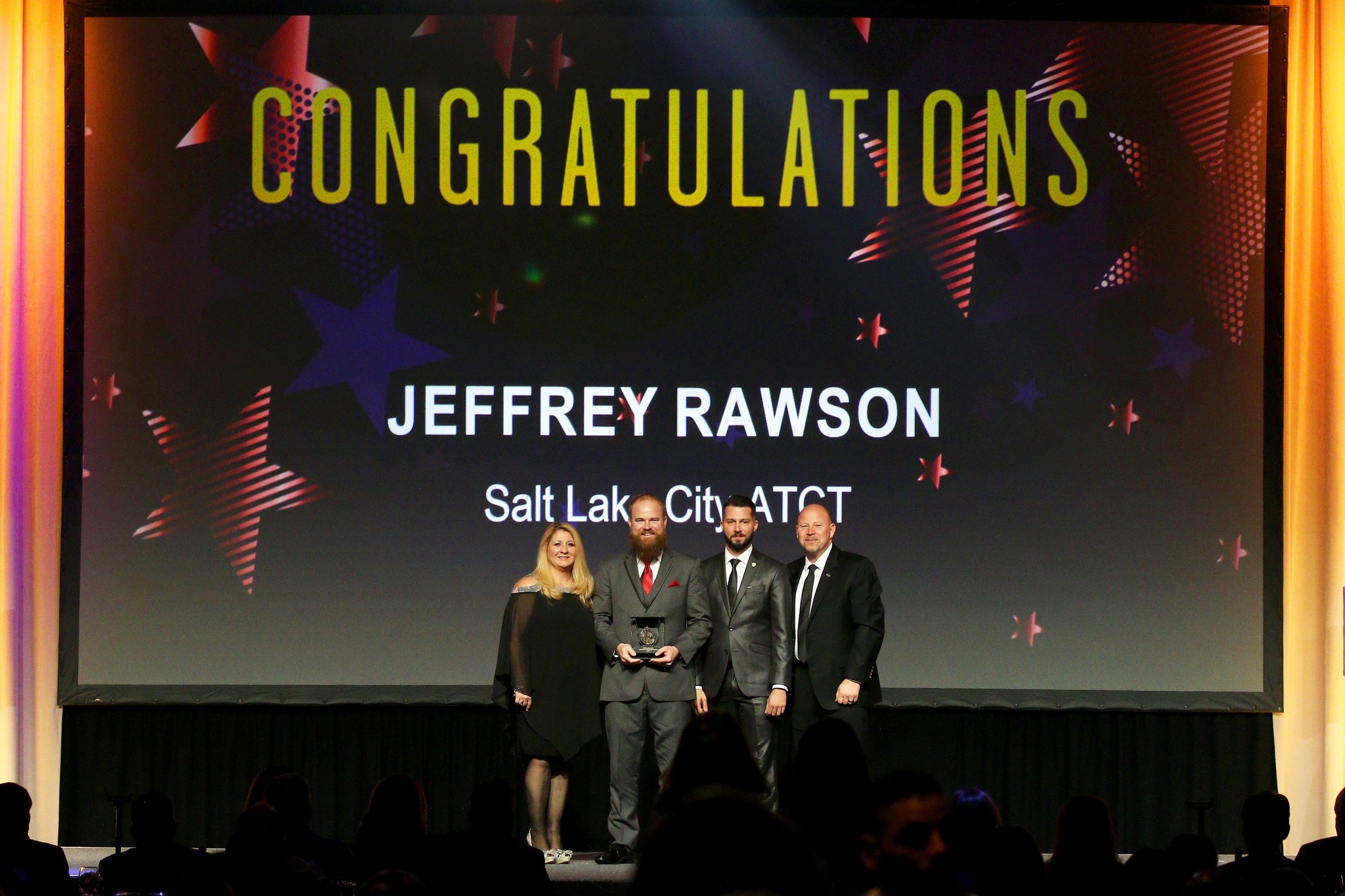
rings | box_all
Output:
[848,699,854,702]
[519,700,523,705]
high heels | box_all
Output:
[526,828,556,864]
[549,846,573,864]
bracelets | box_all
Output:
[848,678,861,685]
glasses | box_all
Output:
[893,815,953,850]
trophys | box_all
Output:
[630,615,666,660]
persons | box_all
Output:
[693,494,794,815]
[591,494,713,863]
[634,709,1344,896]
[0,782,71,896]
[492,522,604,864]
[785,503,885,743]
[98,774,551,884]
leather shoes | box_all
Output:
[595,843,635,864]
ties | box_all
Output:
[639,556,654,595]
[798,564,818,663]
[726,559,741,613]
